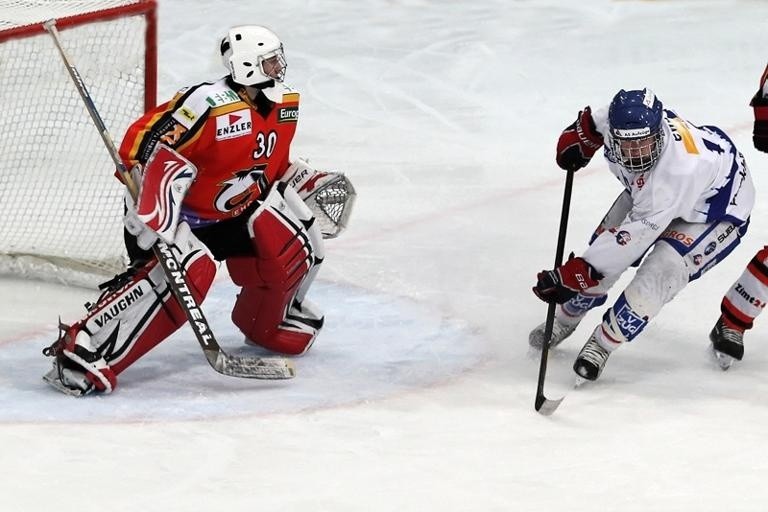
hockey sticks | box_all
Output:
[43,18,295,380]
[535,147,575,416]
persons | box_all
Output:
[709,65,768,361]
[55,25,324,394]
[528,87,756,381]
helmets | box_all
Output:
[608,88,665,173]
[220,26,286,104]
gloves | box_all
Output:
[750,90,768,152]
[533,253,604,305]
[117,163,158,250]
[557,107,602,171]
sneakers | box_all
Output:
[573,325,609,380]
[710,314,745,360]
[530,316,581,351]
